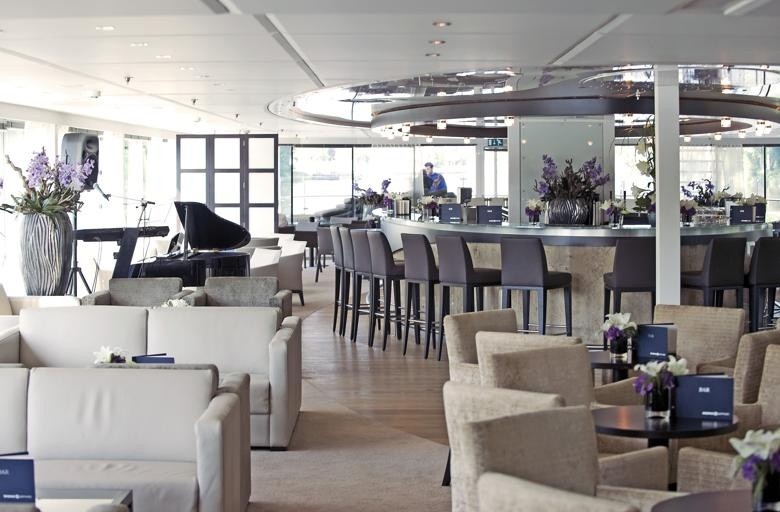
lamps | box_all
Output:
[714,133,723,141]
[750,118,772,138]
[425,135,433,144]
[737,130,746,139]
[720,118,732,128]
[463,137,471,144]
[436,120,447,130]
[683,136,691,143]
[384,117,413,145]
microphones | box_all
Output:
[92,183,109,202]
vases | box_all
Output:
[609,213,624,230]
[20,210,74,296]
[363,204,380,223]
[682,215,691,226]
[422,208,432,219]
[529,214,540,225]
[546,199,591,226]
[647,207,656,226]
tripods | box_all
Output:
[65,191,91,296]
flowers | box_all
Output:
[525,154,612,204]
[683,180,766,205]
[525,199,546,216]
[420,195,438,209]
[680,200,697,217]
[632,136,655,211]
[1,148,97,228]
[351,178,395,207]
[598,198,630,215]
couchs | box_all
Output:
[0,217,335,512]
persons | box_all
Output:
[424,169,441,196]
[423,162,447,194]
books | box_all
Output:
[674,370,735,422]
[0,450,35,503]
[630,320,679,364]
[131,352,175,363]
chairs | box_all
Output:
[399,232,447,358]
[744,236,780,333]
[327,223,420,354]
[601,238,657,350]
[681,237,746,308]
[500,237,576,337]
[434,233,501,361]
[435,306,780,511]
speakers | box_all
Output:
[62,133,99,188]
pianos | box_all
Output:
[74,228,168,241]
[130,203,251,287]
